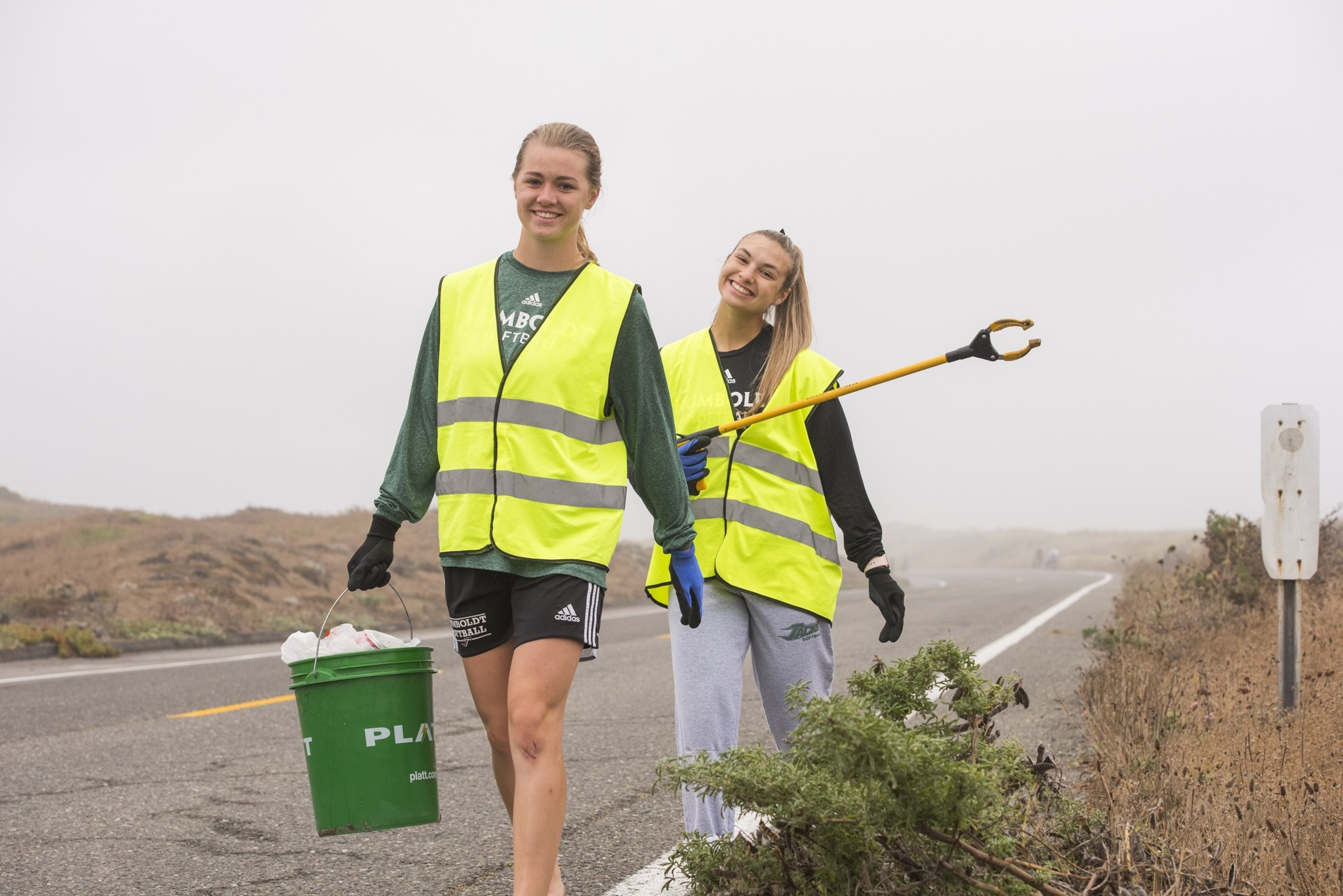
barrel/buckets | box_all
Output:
[286,571,443,836]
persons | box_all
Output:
[649,231,906,846]
[346,123,704,896]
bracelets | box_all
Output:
[864,553,889,573]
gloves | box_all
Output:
[677,435,712,495]
[668,544,705,627]
[346,512,401,592]
[862,555,906,643]
